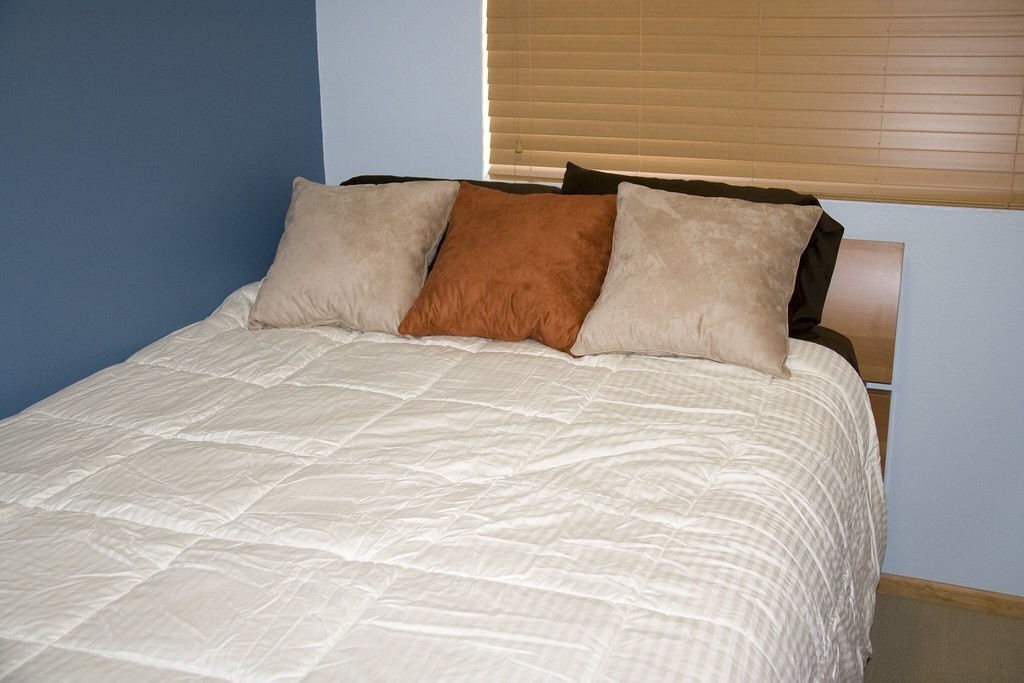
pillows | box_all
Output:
[560,161,846,341]
[248,176,461,338]
[571,183,824,380]
[399,183,617,358]
[339,176,563,274]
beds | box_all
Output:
[0,176,905,683]
